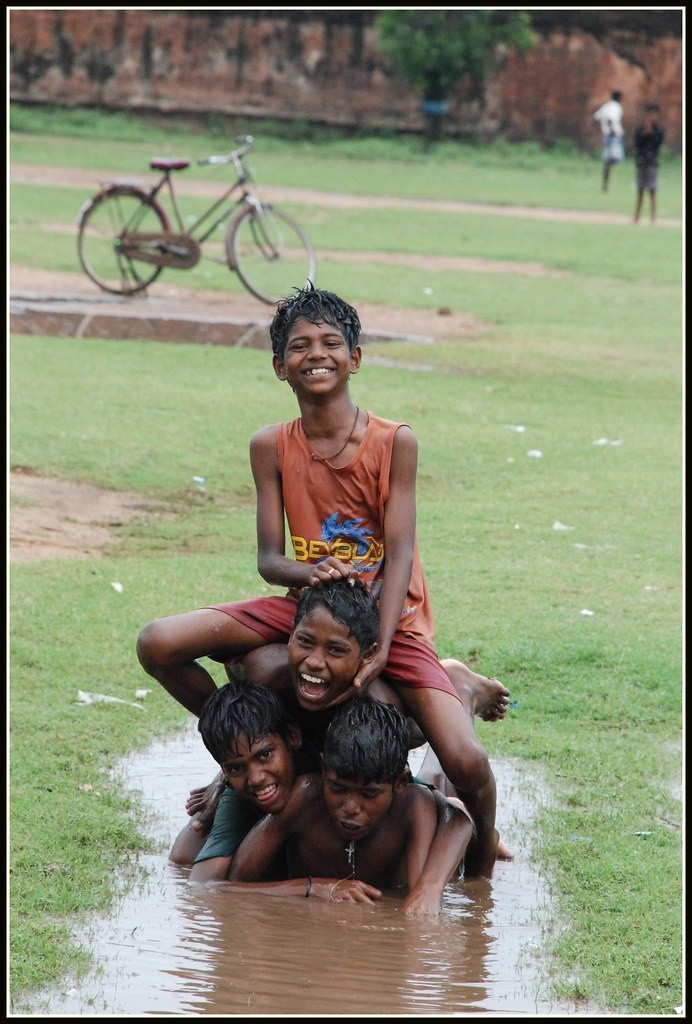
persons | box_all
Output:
[593,90,625,192]
[137,276,500,879]
[632,103,665,223]
[169,574,515,915]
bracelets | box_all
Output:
[305,877,311,897]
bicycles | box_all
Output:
[78,136,318,307]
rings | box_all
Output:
[328,568,334,574]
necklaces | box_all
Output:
[308,406,360,461]
[343,838,359,863]
